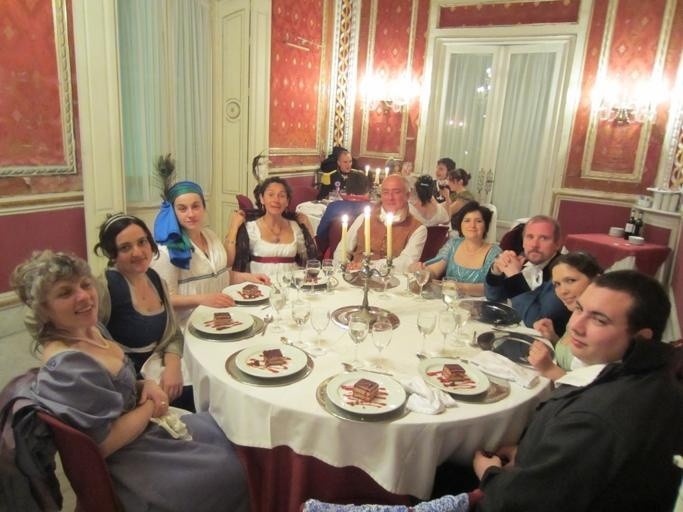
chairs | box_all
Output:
[37,406,123,512]
[418,225,449,263]
[323,216,356,260]
[234,194,255,211]
[497,224,527,255]
[669,336,682,377]
[299,485,484,512]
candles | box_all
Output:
[365,164,370,176]
[373,168,382,185]
[385,213,394,259]
[362,206,372,252]
[384,165,390,178]
[341,215,350,263]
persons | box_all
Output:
[473,269,683,510]
[226,177,317,278]
[16,250,249,511]
[93,213,193,411]
[152,180,271,333]
[528,252,600,380]
[316,150,502,295]
[483,216,570,336]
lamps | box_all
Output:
[360,72,417,113]
[595,75,657,126]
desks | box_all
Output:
[565,233,671,281]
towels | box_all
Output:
[400,375,457,415]
[471,350,541,389]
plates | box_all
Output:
[222,283,272,302]
[236,343,307,379]
[327,371,407,415]
[460,298,521,326]
[609,227,623,238]
[478,332,556,367]
[418,358,492,397]
[191,309,254,333]
[629,235,644,243]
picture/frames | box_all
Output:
[0,0,76,175]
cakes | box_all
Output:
[214,312,232,326]
[243,285,258,295]
[353,378,379,402]
[264,349,285,366]
[443,364,466,381]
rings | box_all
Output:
[162,401,166,404]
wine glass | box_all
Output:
[436,181,447,201]
[401,161,412,178]
[271,260,470,366]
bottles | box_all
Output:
[623,211,643,238]
[639,196,650,207]
